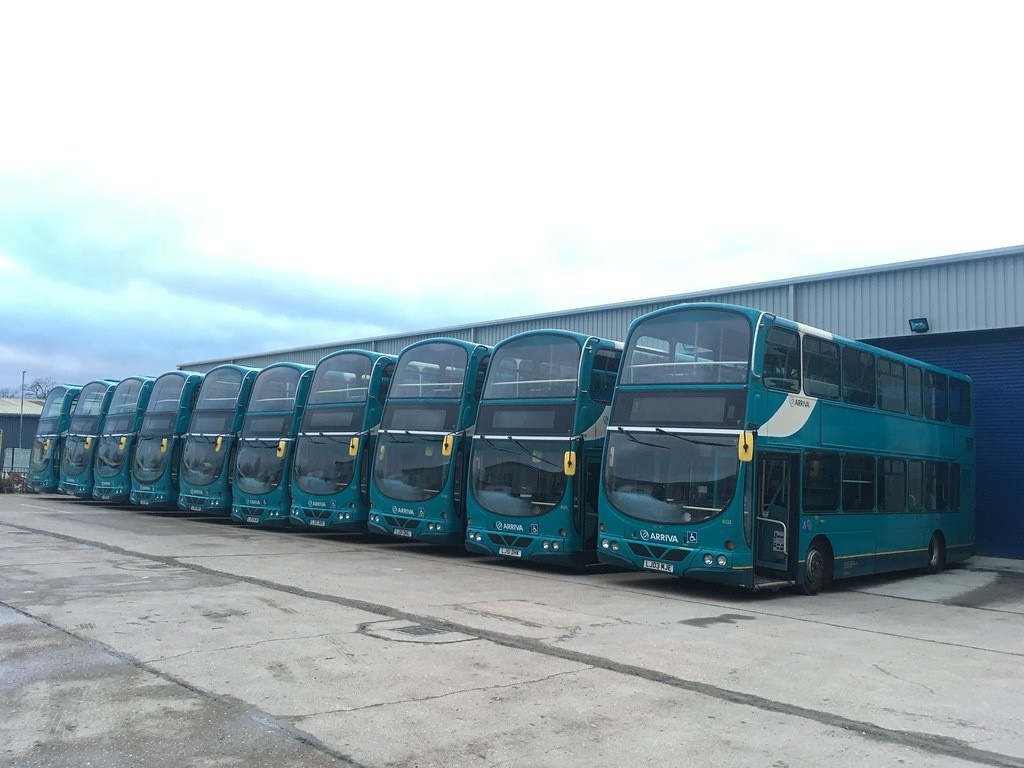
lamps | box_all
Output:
[908,318,930,333]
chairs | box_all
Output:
[909,491,949,510]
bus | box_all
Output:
[26,383,83,494]
[230,362,436,529]
[289,348,560,534]
[56,378,139,498]
[90,376,243,502]
[597,302,977,596]
[128,370,298,508]
[366,337,619,549]
[463,329,747,567]
[177,364,393,516]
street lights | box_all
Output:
[18,370,28,448]
